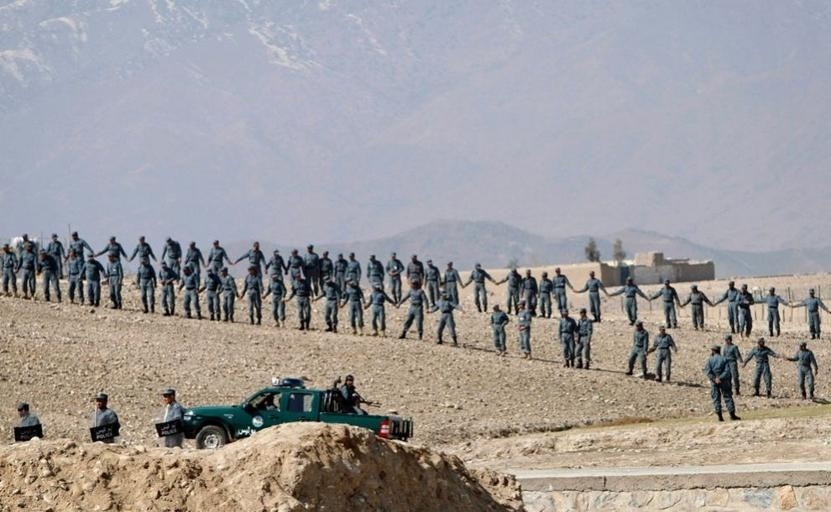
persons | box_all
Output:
[0,230,831,403]
[16,402,39,429]
[159,389,184,448]
[266,395,279,412]
[97,392,114,441]
[704,343,742,423]
[340,372,367,416]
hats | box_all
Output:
[92,391,109,401]
[493,304,499,310]
[17,401,31,412]
[2,230,561,285]
[562,309,568,314]
[159,387,177,397]
[710,335,807,353]
[659,326,665,330]
[636,320,644,325]
[518,300,526,306]
[579,308,586,313]
[344,374,356,381]
[590,270,814,295]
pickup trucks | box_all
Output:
[178,378,414,452]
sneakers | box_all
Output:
[655,374,663,381]
[571,359,575,367]
[666,376,671,382]
[730,410,743,420]
[584,360,590,369]
[753,388,760,396]
[767,390,772,397]
[562,358,570,368]
[810,391,814,399]
[576,358,583,369]
[802,392,807,401]
[624,368,634,375]
[735,386,740,394]
[643,371,648,379]
[715,410,725,422]
